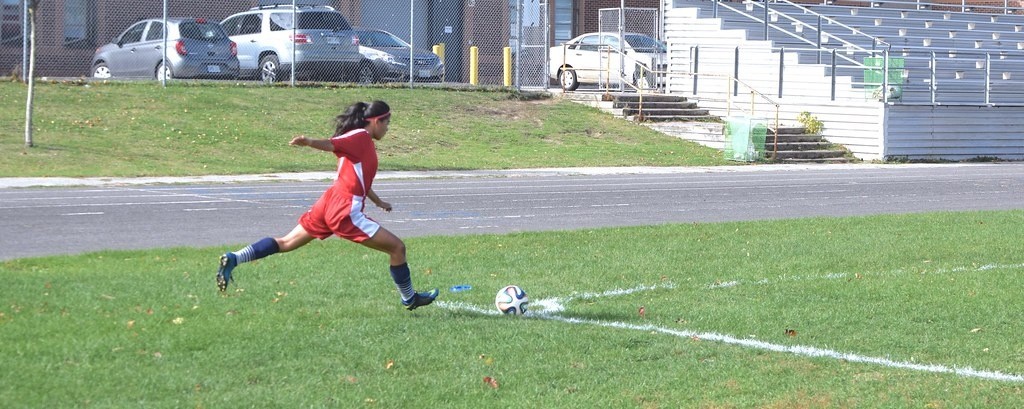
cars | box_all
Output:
[353,27,444,82]
[547,32,668,90]
[92,17,241,81]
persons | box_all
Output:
[215,104,438,312]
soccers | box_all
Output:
[495,285,530,316]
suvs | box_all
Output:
[218,3,361,82]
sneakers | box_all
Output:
[402,288,439,311]
[216,251,236,292]
